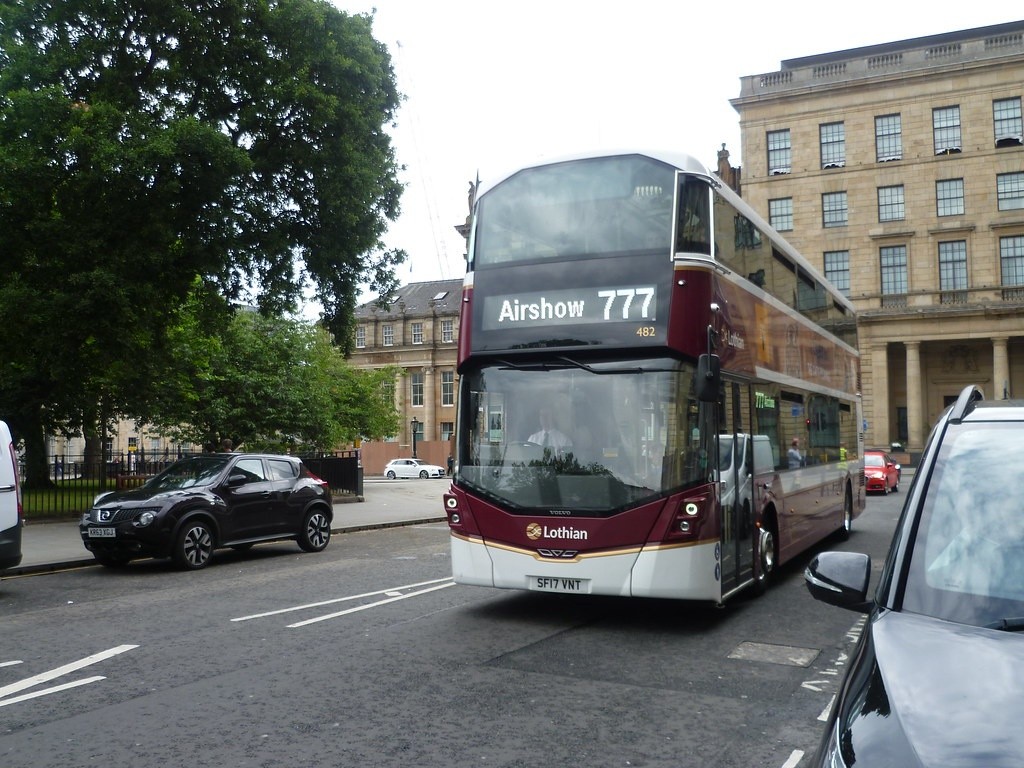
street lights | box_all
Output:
[411,415,420,458]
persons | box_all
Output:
[788,438,805,468]
[468,181,475,215]
[223,439,233,453]
[840,440,848,461]
[717,143,731,184]
[447,452,454,476]
[523,408,573,454]
[206,443,216,453]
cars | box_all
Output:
[383,458,446,479]
[862,451,902,494]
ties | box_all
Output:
[541,432,550,447]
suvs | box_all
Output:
[72,452,335,570]
[803,384,1024,768]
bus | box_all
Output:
[454,148,873,608]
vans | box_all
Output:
[0,421,25,568]
